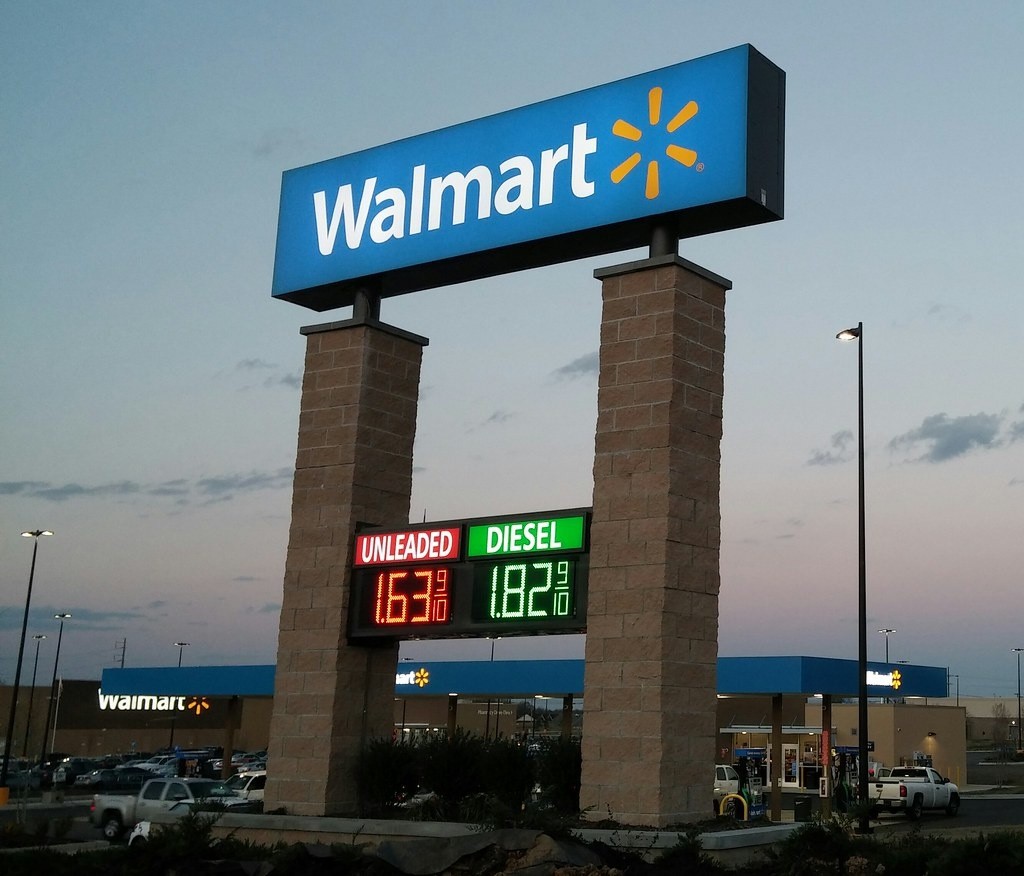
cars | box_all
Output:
[223,771,266,807]
[0,747,267,793]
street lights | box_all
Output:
[834,319,871,814]
[877,628,897,663]
[1011,647,1024,753]
[484,633,503,745]
[39,612,73,768]
[22,634,47,757]
[0,530,55,784]
[169,641,191,747]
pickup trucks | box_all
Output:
[713,764,741,812]
[86,776,250,843]
[855,765,962,823]
